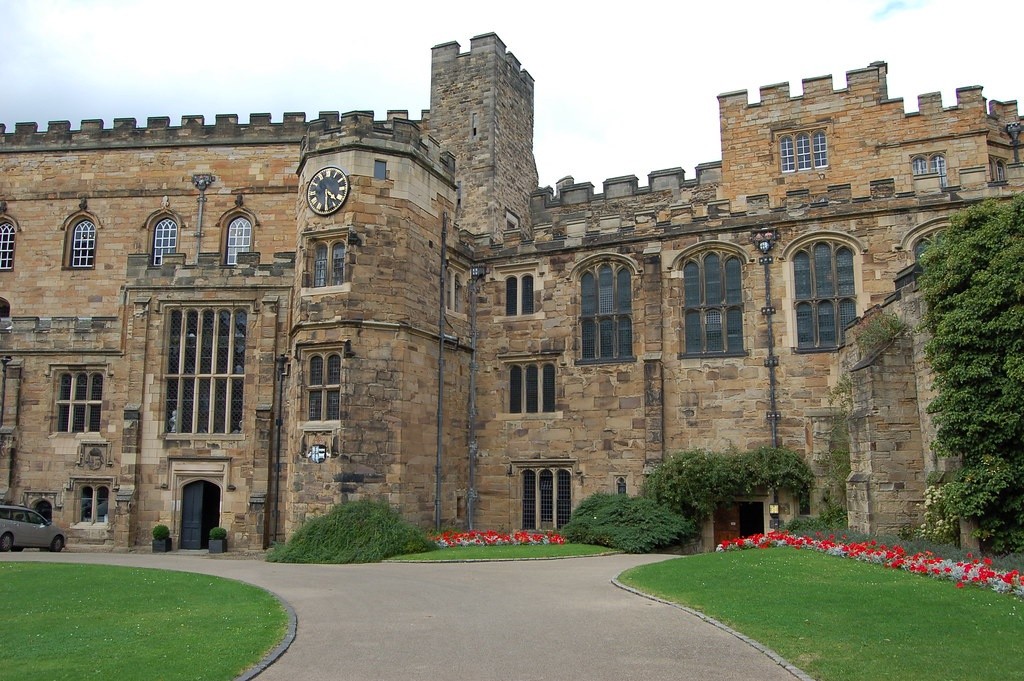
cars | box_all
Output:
[1,504,67,553]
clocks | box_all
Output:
[307,166,349,217]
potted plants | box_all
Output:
[209,527,226,554]
[151,525,171,553]
